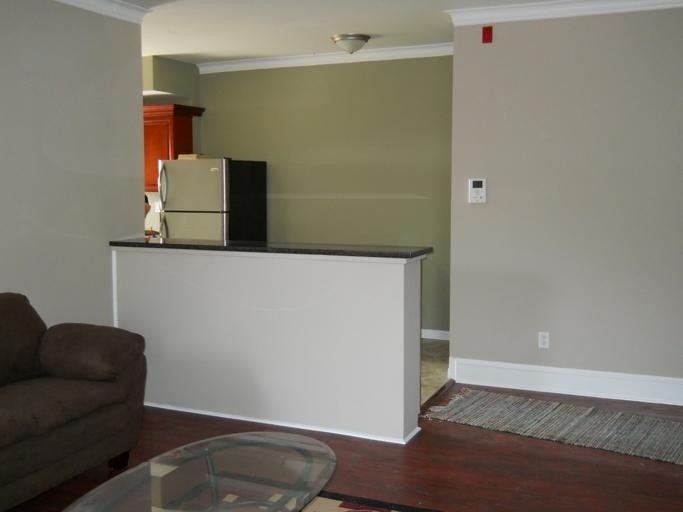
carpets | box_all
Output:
[419,387,683,466]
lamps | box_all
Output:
[331,33,372,57]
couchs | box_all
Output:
[0,293,147,512]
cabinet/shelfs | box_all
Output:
[144,103,193,193]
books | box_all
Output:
[178,153,211,160]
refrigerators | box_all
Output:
[156,158,270,241]
